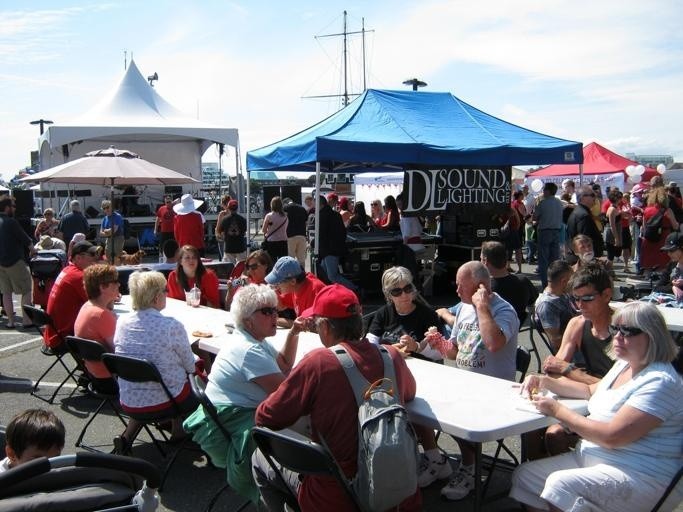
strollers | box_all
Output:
[26,246,70,316]
[0,421,160,510]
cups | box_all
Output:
[184,292,190,304]
[190,287,201,307]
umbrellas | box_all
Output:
[16,143,202,265]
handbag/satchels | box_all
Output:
[261,241,269,252]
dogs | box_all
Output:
[120,249,147,265]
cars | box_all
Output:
[216,195,260,214]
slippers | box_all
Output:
[4,323,35,330]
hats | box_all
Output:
[264,255,302,284]
[300,282,363,319]
[71,240,103,258]
[227,200,239,207]
[630,184,644,194]
[664,181,680,188]
[646,175,665,188]
[37,235,56,250]
[71,233,86,242]
[660,231,683,251]
[172,194,204,216]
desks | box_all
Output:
[198,329,589,512]
[110,293,285,357]
[608,300,683,332]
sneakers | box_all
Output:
[418,454,454,487]
[508,257,540,275]
[112,435,132,457]
[440,465,476,500]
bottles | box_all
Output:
[132,475,162,511]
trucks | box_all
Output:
[28,183,108,218]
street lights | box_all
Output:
[29,117,52,133]
[401,77,430,93]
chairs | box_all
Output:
[535,317,556,358]
[188,371,261,512]
[519,312,541,373]
[64,335,156,454]
[435,344,531,512]
[101,352,216,494]
[22,304,95,405]
[249,426,364,512]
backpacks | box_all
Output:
[310,342,419,512]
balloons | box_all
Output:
[622,164,644,184]
[655,163,667,175]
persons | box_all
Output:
[1,407,65,475]
[41,240,414,512]
[367,241,522,500]
[510,260,683,512]
[505,175,682,352]
[0,174,397,329]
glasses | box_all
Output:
[254,307,279,318]
[607,324,644,338]
[389,283,414,297]
[245,262,262,271]
[573,291,600,301]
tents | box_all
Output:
[524,142,659,192]
[238,88,586,280]
